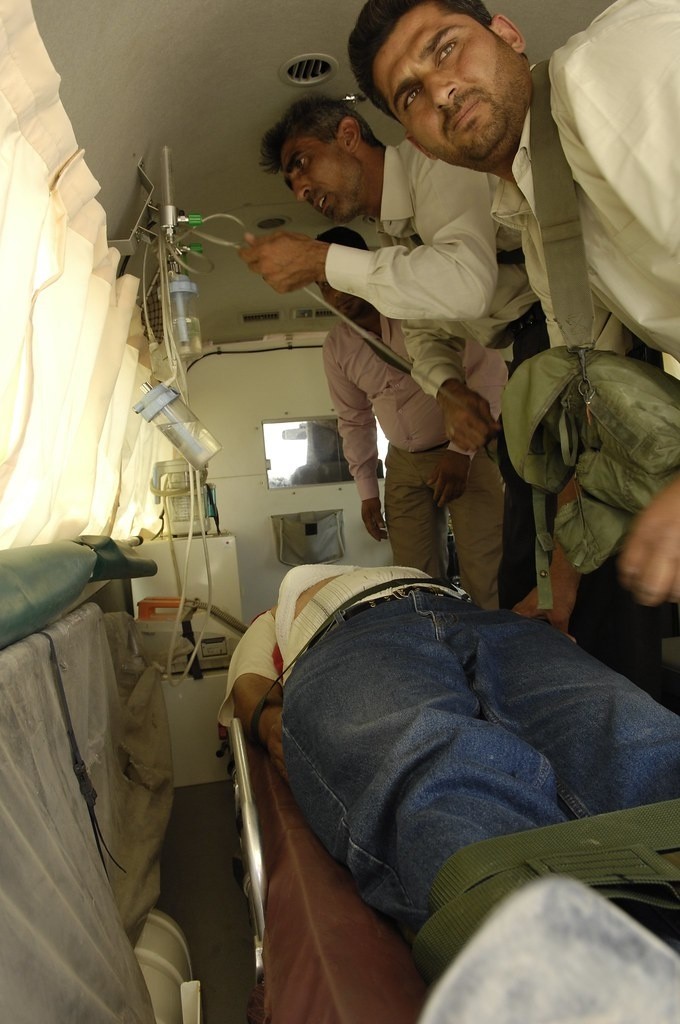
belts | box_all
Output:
[308,585,459,648]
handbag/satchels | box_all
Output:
[499,347,680,574]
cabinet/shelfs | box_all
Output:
[129,535,244,787]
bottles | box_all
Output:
[158,271,203,357]
[135,382,220,468]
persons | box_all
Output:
[240,94,552,611]
[347,0,680,717]
[218,564,680,1024]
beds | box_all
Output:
[217,718,427,1024]
